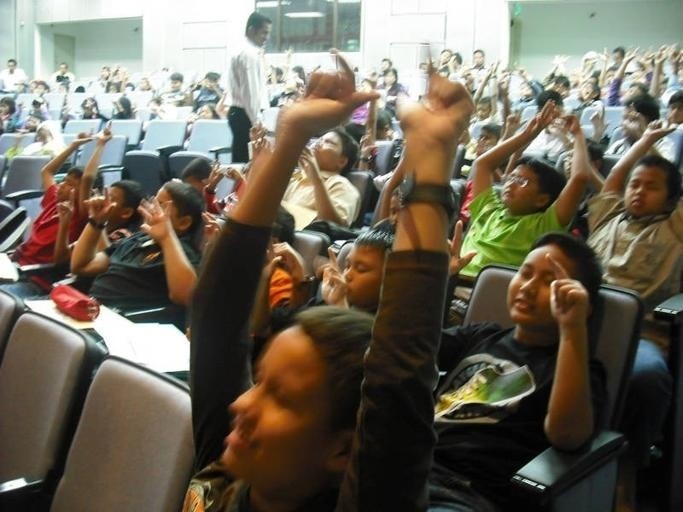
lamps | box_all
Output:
[282,0,329,19]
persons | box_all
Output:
[225,10,273,162]
[1,42,682,512]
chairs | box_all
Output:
[0,70,682,512]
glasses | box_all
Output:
[500,172,535,188]
